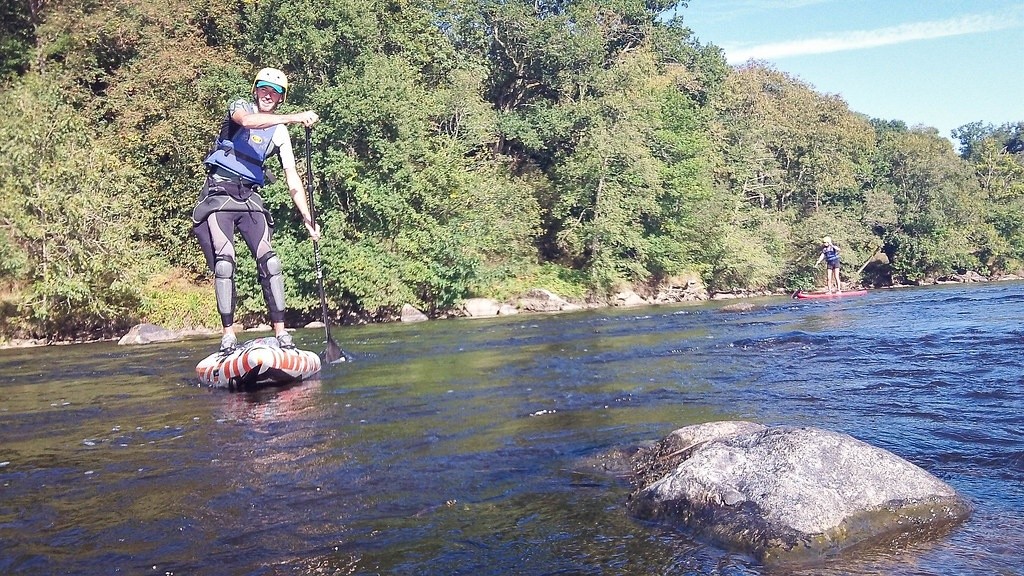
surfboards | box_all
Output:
[195,344,321,389]
[798,290,867,300]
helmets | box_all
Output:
[251,68,288,104]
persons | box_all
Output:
[192,68,321,363]
[813,236,841,294]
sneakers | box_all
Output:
[219,334,237,351]
[278,331,295,350]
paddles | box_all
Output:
[792,261,820,299]
[305,126,347,361]
